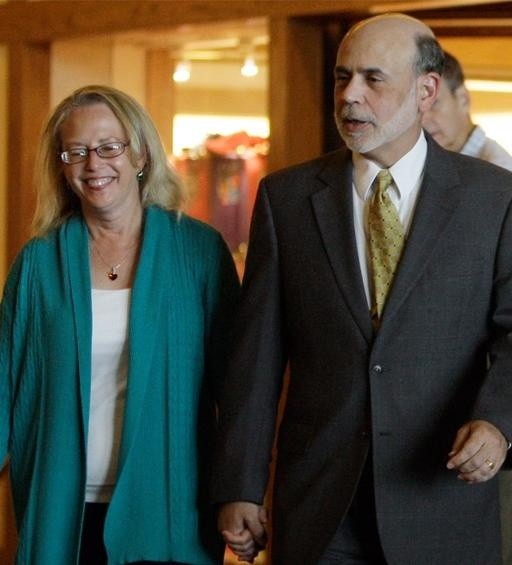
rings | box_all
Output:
[486,458,497,471]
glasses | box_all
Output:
[53,140,131,166]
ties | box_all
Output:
[364,168,410,334]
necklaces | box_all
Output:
[87,236,140,285]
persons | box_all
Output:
[420,49,512,174]
[0,82,270,565]
[199,8,512,565]
